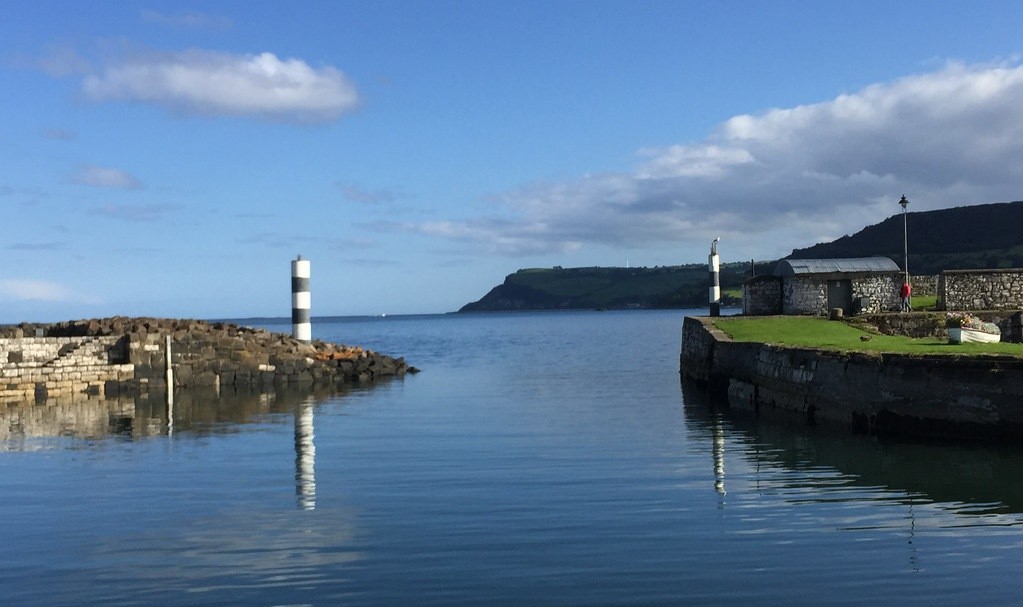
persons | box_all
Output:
[900,283,913,313]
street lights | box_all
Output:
[897,193,912,312]
